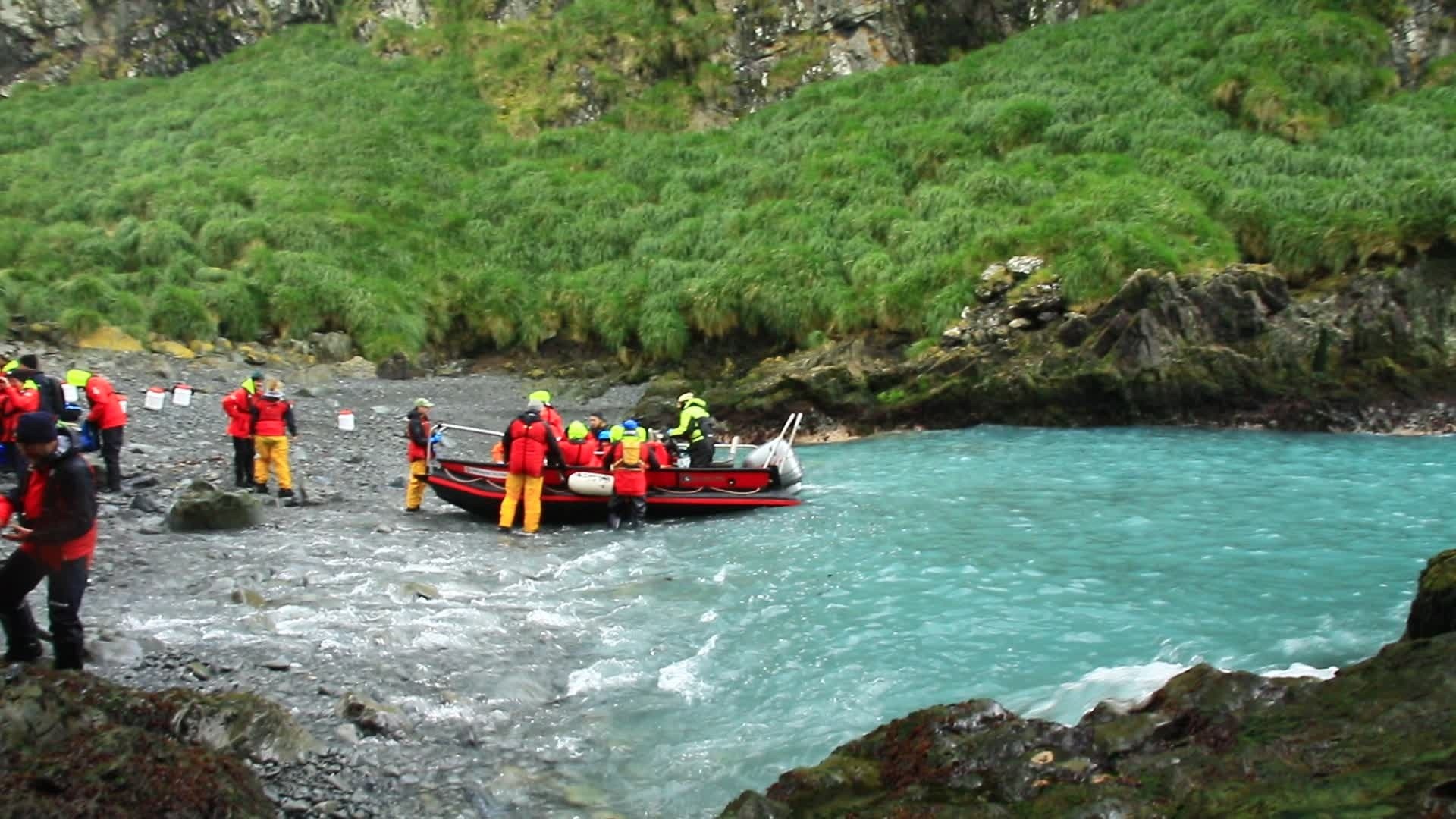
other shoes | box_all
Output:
[407,506,419,512]
[236,477,244,487]
[258,483,269,493]
[278,488,295,498]
[3,639,42,664]
[103,487,120,493]
[498,526,510,533]
[246,479,255,486]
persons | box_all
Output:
[530,391,668,465]
[65,369,128,492]
[223,371,264,487]
[405,398,443,511]
[0,350,66,468]
[666,392,715,468]
[604,419,660,530]
[249,379,298,498]
[0,412,98,668]
[498,399,564,535]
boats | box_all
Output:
[414,422,803,523]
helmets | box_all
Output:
[679,392,693,402]
[624,420,638,430]
[529,391,551,403]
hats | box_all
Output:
[592,411,606,422]
[267,379,285,389]
[528,399,544,413]
[251,369,264,379]
[9,369,28,383]
[17,413,56,444]
[415,398,435,408]
[19,355,39,369]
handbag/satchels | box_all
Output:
[80,421,101,452]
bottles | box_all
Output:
[116,394,127,414]
[145,386,165,410]
[339,410,355,431]
[173,385,193,407]
[61,381,79,403]
